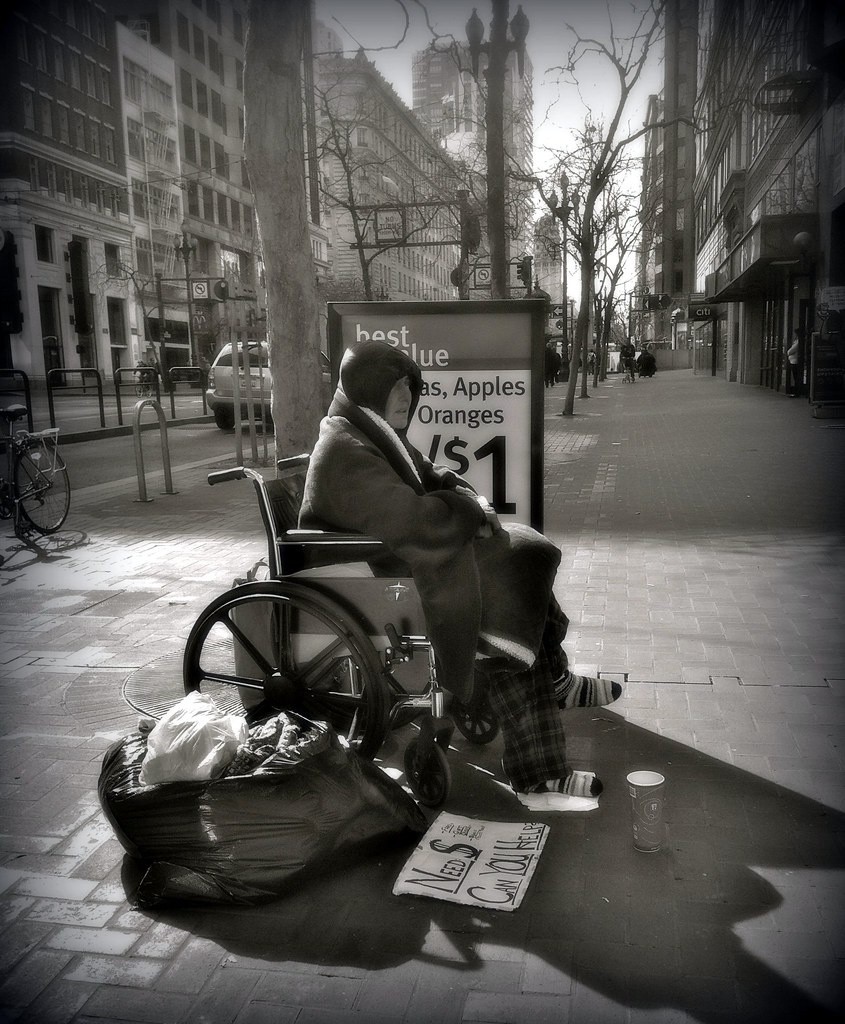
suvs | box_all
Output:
[206,341,332,430]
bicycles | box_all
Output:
[0,404,71,535]
[132,374,152,398]
[622,357,636,383]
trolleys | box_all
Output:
[184,451,500,809]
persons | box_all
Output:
[637,346,656,378]
[544,343,561,388]
[297,339,625,799]
[786,328,799,397]
[133,357,161,392]
[620,337,635,382]
[586,349,596,375]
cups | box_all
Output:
[626,770,665,854]
